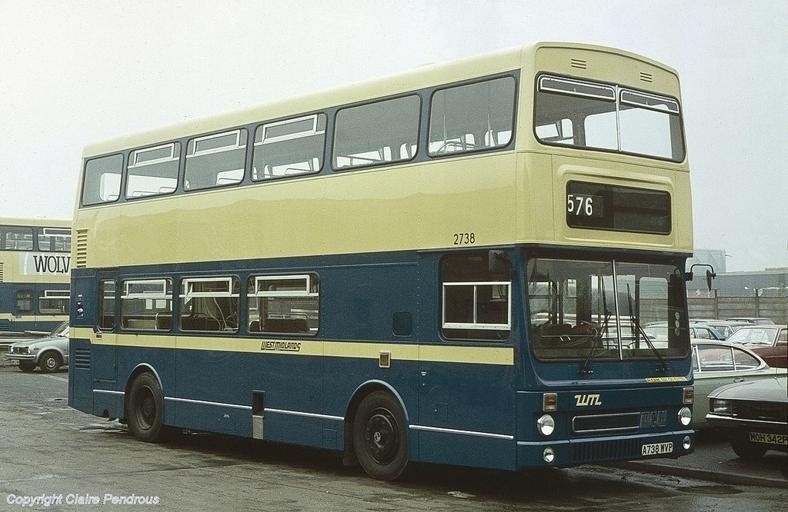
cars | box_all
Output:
[530,313,788,460]
[7,321,70,373]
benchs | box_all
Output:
[104,311,309,338]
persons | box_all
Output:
[452,286,493,339]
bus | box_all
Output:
[67,40,715,484]
[0,217,72,332]
